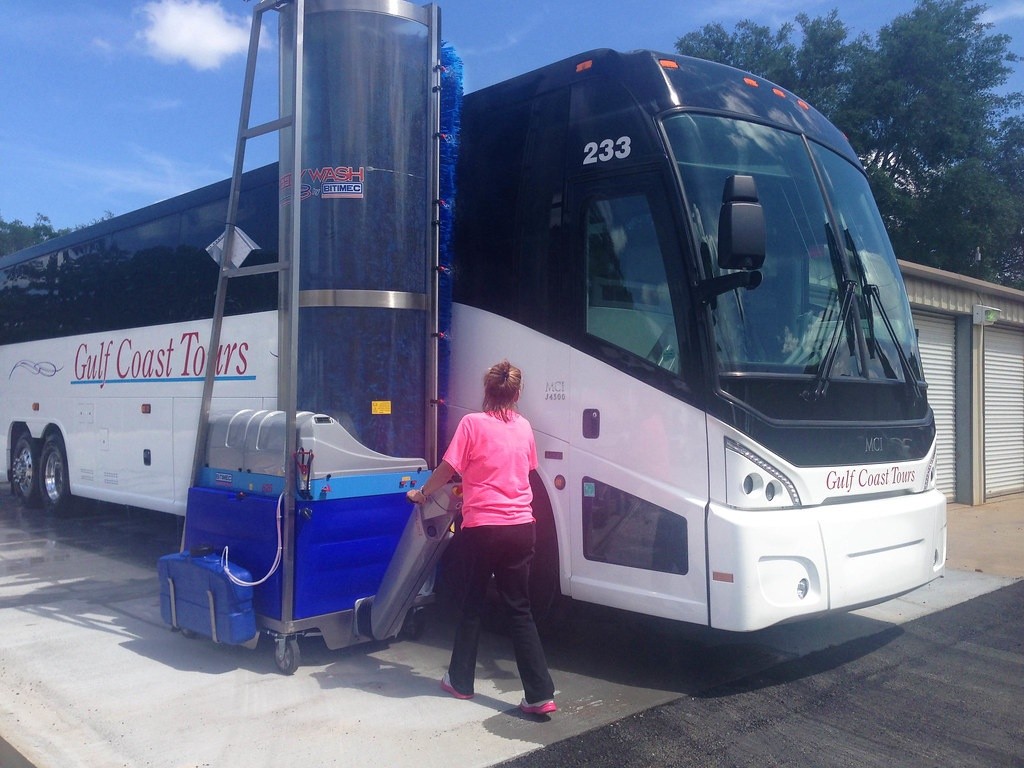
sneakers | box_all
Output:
[520,695,555,714]
[440,671,474,697]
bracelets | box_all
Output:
[419,487,432,499]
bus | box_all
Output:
[5,44,946,644]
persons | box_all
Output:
[407,360,560,715]
